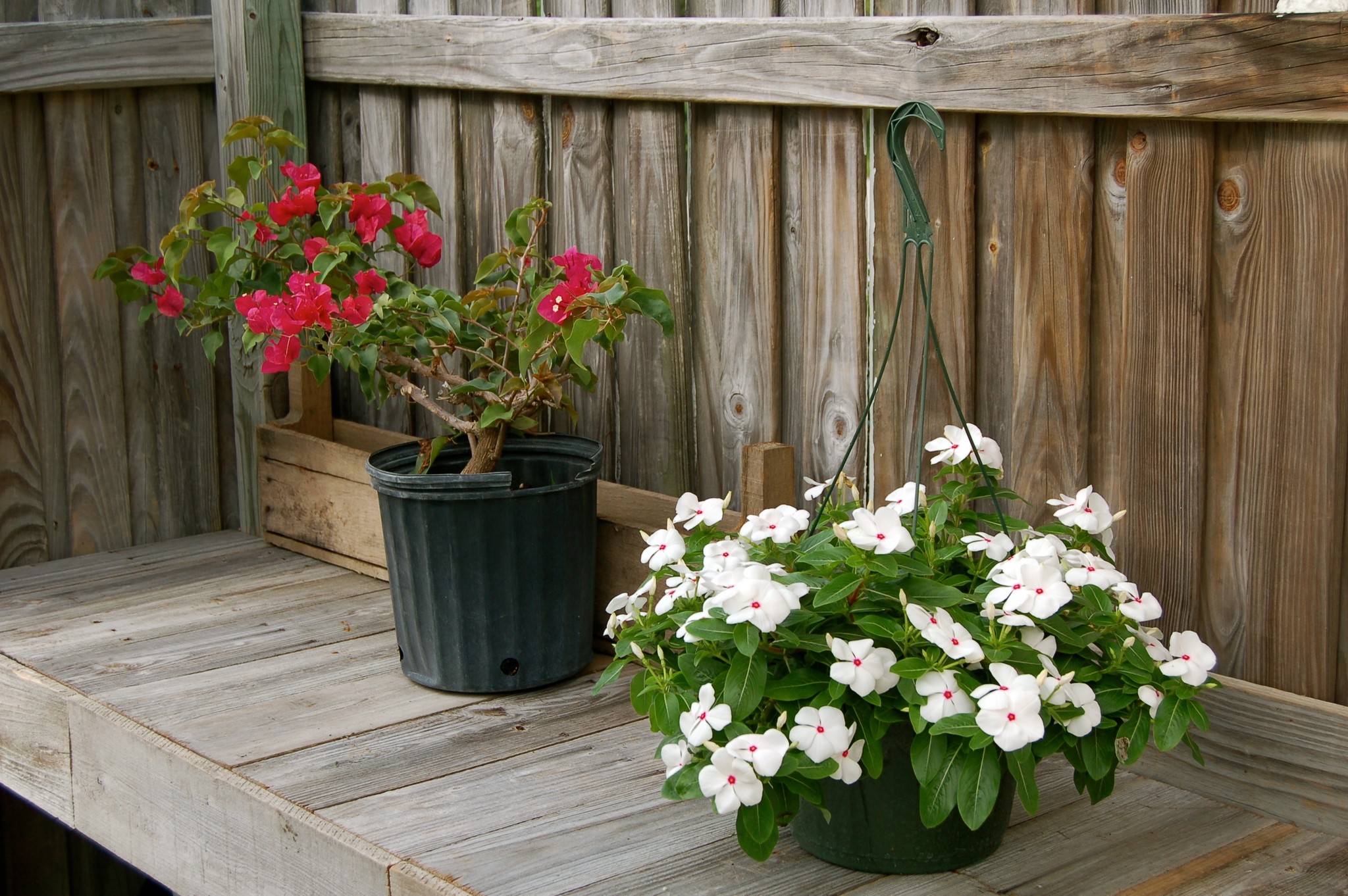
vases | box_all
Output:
[374,429,601,693]
[790,704,1036,881]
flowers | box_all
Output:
[98,116,669,467]
[605,420,1216,862]
